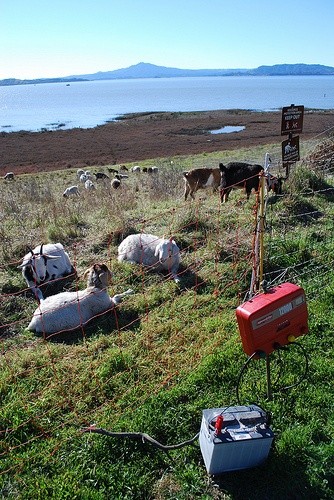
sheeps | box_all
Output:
[117,233,180,283]
[17,242,72,304]
[24,261,133,333]
[182,162,283,204]
[63,185,80,199]
[4,172,15,181]
[76,165,159,189]
